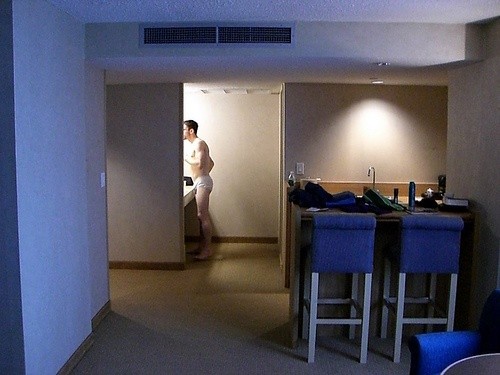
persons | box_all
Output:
[183,120,214,259]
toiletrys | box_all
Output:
[394,188,398,204]
[409,181,415,209]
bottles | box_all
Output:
[409,182,415,212]
[394,188,398,204]
[288,171,295,192]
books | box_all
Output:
[442,195,468,206]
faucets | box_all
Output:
[368,166,375,189]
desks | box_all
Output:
[289,193,475,349]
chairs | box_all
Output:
[407,329,482,375]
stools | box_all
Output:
[302,211,376,365]
[381,215,465,364]
